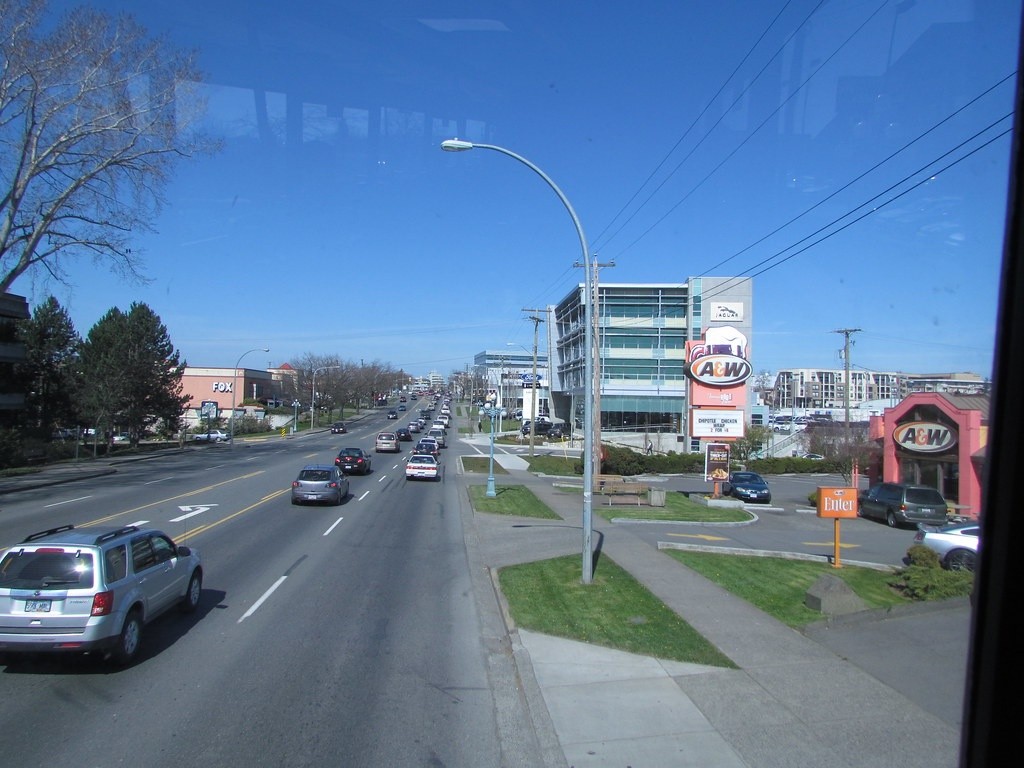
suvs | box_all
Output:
[857,481,947,526]
[1,523,202,666]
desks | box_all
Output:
[948,505,974,524]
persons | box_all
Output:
[478,422,482,432]
[646,440,653,455]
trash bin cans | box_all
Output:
[649,486,666,507]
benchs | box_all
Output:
[591,473,623,497]
[947,513,970,522]
[602,477,649,507]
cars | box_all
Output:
[487,408,523,421]
[722,471,771,504]
[913,521,980,574]
[802,453,824,460]
[769,414,832,433]
[291,463,349,506]
[52,428,155,441]
[331,422,348,434]
[191,430,230,442]
[376,390,454,481]
[335,447,372,476]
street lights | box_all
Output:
[230,348,268,446]
[291,399,301,431]
[474,363,502,410]
[310,366,342,428]
[507,341,535,456]
[479,392,507,497]
[440,133,596,587]
[203,399,216,442]
